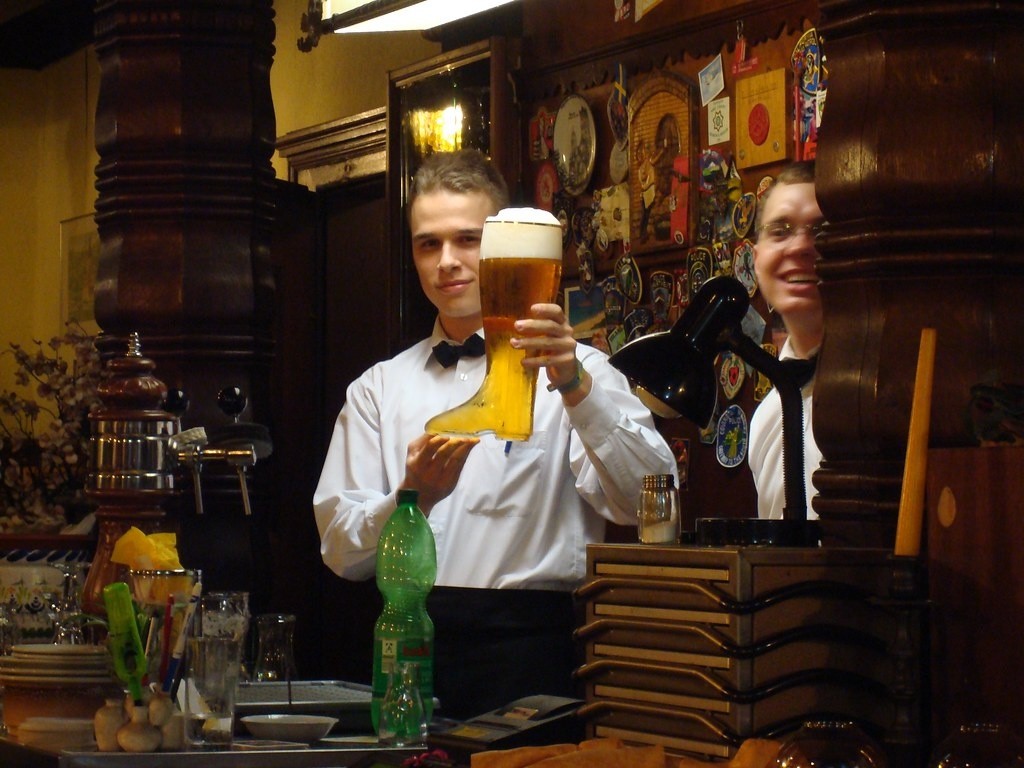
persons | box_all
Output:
[312,149,681,717]
[748,159,824,521]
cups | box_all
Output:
[425,220,563,444]
[253,614,297,680]
[183,591,248,748]
[131,569,203,656]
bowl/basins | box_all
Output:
[240,714,340,744]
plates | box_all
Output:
[0,644,113,684]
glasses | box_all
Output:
[753,220,825,246]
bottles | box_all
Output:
[102,581,146,701]
[369,489,435,745]
[639,473,679,544]
[55,571,84,643]
[81,505,130,620]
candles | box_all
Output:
[894,325,939,556]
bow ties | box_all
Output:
[434,332,485,368]
[778,351,819,388]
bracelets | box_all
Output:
[547,361,582,392]
